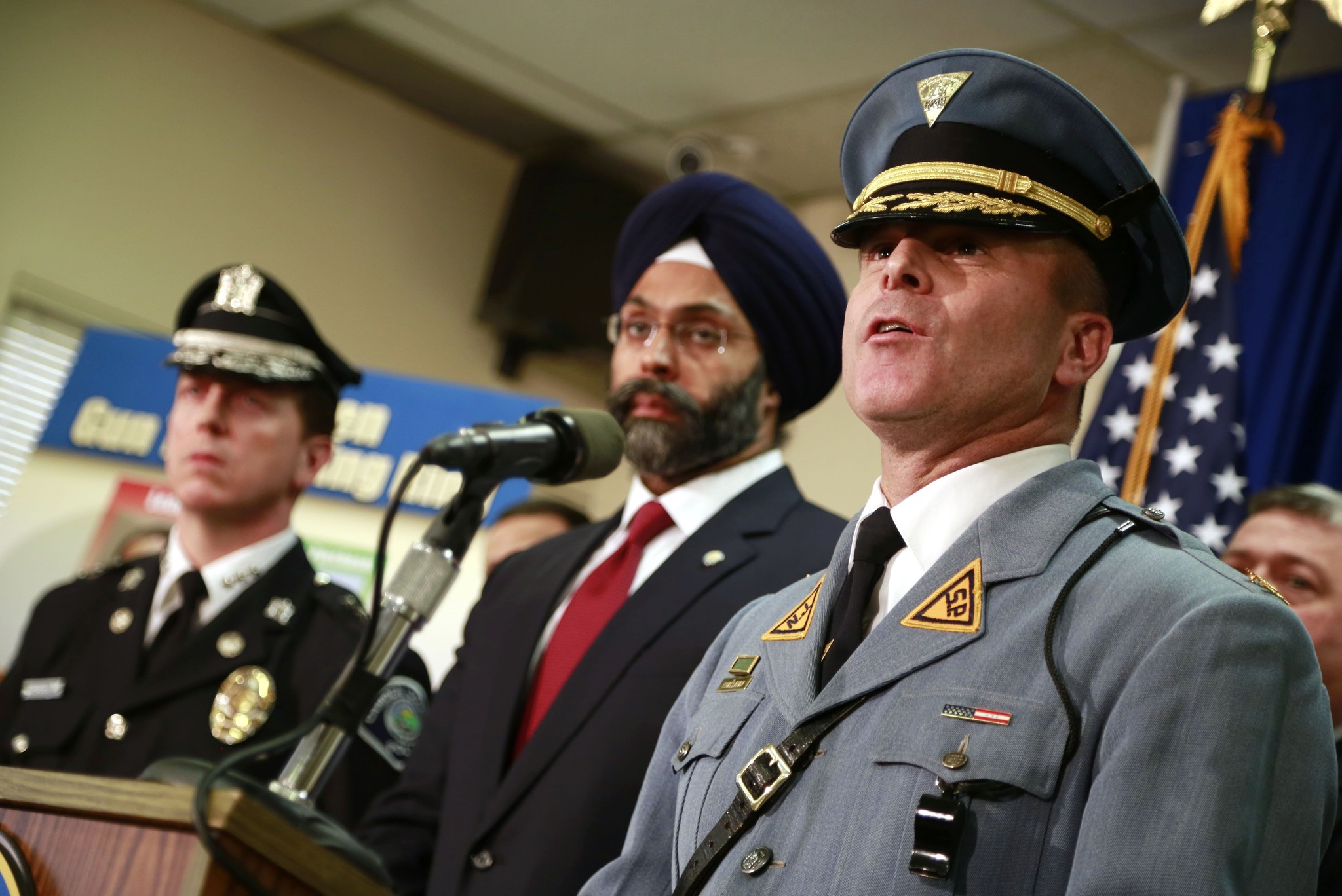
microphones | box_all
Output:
[422,408,622,490]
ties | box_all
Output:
[135,571,215,679]
[499,502,676,776]
[812,509,905,692]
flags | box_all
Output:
[1082,97,1259,552]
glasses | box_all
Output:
[604,310,757,350]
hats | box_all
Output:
[162,262,362,399]
[833,49,1192,338]
[612,171,844,423]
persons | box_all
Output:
[571,48,1342,895]
[1,260,433,836]
[358,169,849,895]
[1220,479,1340,895]
[480,497,591,583]
